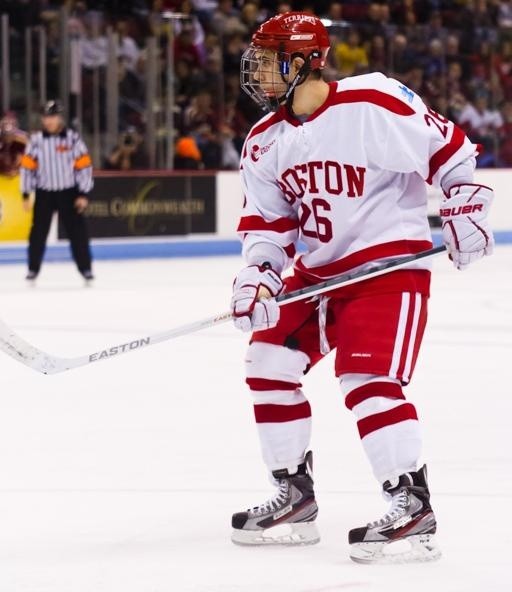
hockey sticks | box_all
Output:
[2,245,445,376]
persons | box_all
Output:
[19,99,96,282]
[230,10,497,546]
[1,1,512,173]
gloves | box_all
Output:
[230,241,289,332]
[439,183,495,272]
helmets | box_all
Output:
[239,11,332,113]
[38,98,67,136]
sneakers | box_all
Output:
[27,271,37,279]
[83,269,93,280]
[231,450,318,531]
[348,468,436,543]
